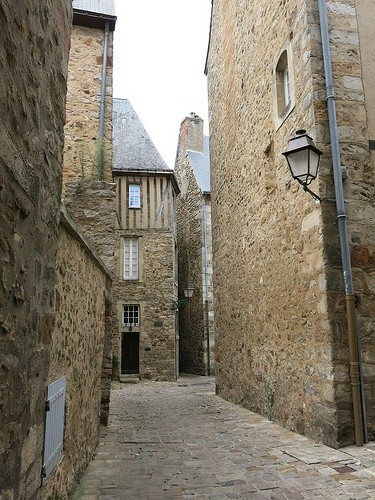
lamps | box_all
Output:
[281,129,336,205]
[171,281,196,311]
